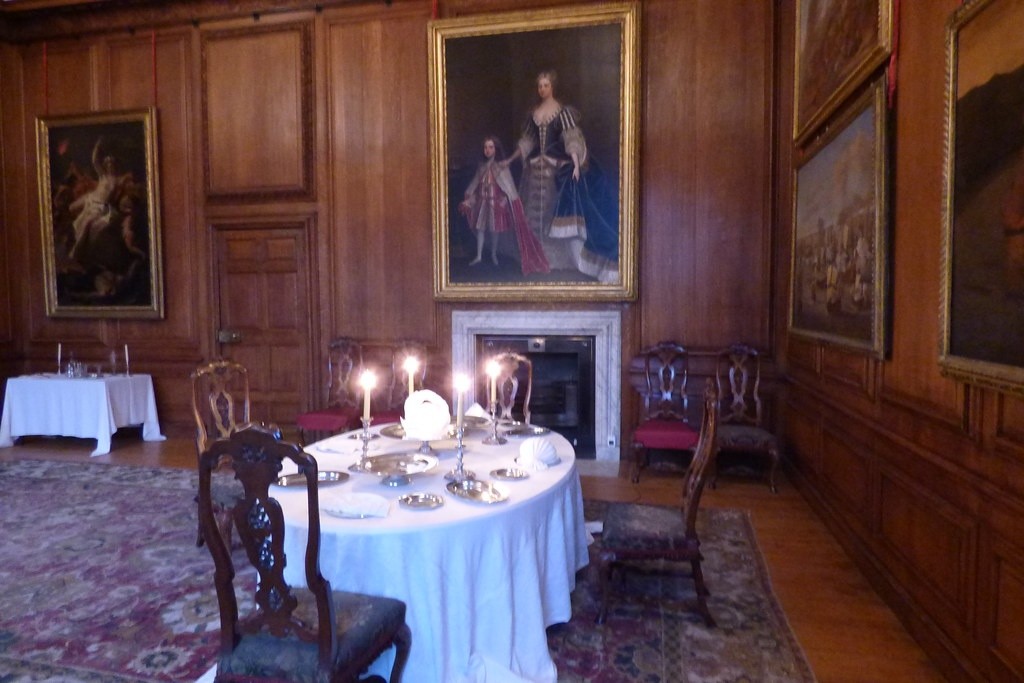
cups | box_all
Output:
[68,362,86,377]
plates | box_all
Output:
[496,468,529,479]
[399,492,443,509]
[455,413,490,428]
[380,424,405,439]
[445,481,510,502]
[348,453,438,473]
[504,420,551,434]
[278,470,350,485]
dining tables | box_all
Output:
[256,417,593,683]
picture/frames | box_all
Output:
[426,0,644,303]
[33,103,167,322]
[936,0,1024,400]
[790,0,892,148]
[783,84,892,362]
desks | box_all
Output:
[0,373,168,455]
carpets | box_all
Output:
[0,452,819,683]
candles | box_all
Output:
[403,354,423,397]
[483,359,502,401]
[58,343,61,366]
[359,371,378,421]
[452,372,471,427]
[125,344,128,363]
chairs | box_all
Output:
[188,357,282,556]
[484,350,533,424]
[198,422,412,683]
[624,341,779,492]
[298,335,429,448]
[595,376,722,629]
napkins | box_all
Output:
[315,437,382,455]
[318,491,391,519]
[464,402,492,427]
